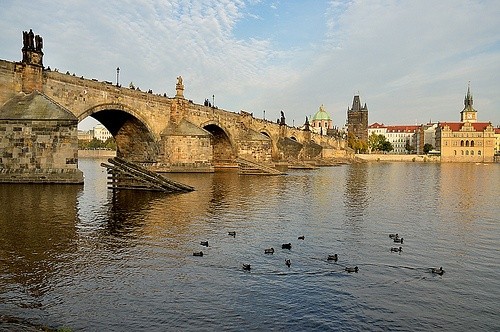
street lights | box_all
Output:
[116,67,120,86]
[263,110,265,120]
[293,120,294,127]
[212,94,214,107]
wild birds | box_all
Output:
[285,259,291,265]
[388,233,404,242]
[432,267,444,275]
[281,243,291,247]
[201,241,208,244]
[228,231,236,235]
[298,236,304,239]
[264,248,274,253]
[242,264,250,269]
[328,254,337,259]
[345,266,359,272]
[391,247,402,251]
[193,251,203,255]
